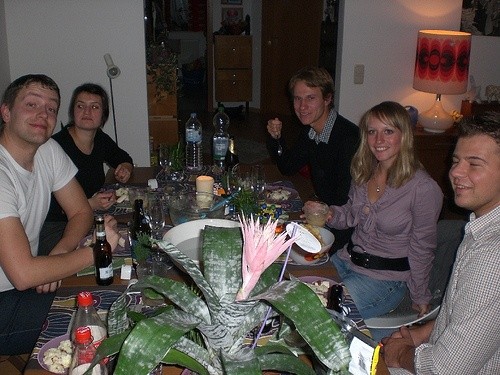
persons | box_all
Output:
[0,75,120,356]
[266,64,360,257]
[38,84,134,255]
[300,101,444,321]
[380,102,500,375]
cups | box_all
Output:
[248,165,266,194]
[127,187,165,234]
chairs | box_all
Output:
[178,56,204,96]
[365,220,466,329]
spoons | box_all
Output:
[275,134,283,156]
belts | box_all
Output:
[347,240,410,271]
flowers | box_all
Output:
[82,207,353,375]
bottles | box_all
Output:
[69,327,110,375]
[128,198,152,267]
[225,136,239,173]
[185,114,202,169]
[213,105,231,162]
[325,284,343,316]
[69,291,109,370]
[93,216,113,285]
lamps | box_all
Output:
[413,28,472,134]
[104,53,122,145]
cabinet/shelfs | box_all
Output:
[412,128,465,216]
[213,34,254,115]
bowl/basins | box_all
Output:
[168,191,226,229]
[284,224,335,264]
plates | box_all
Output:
[37,333,73,375]
[262,184,299,202]
[296,275,342,299]
[77,229,133,257]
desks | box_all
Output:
[23,168,391,375]
[147,69,178,149]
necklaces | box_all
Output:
[376,187,379,191]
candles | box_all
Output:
[196,176,214,207]
